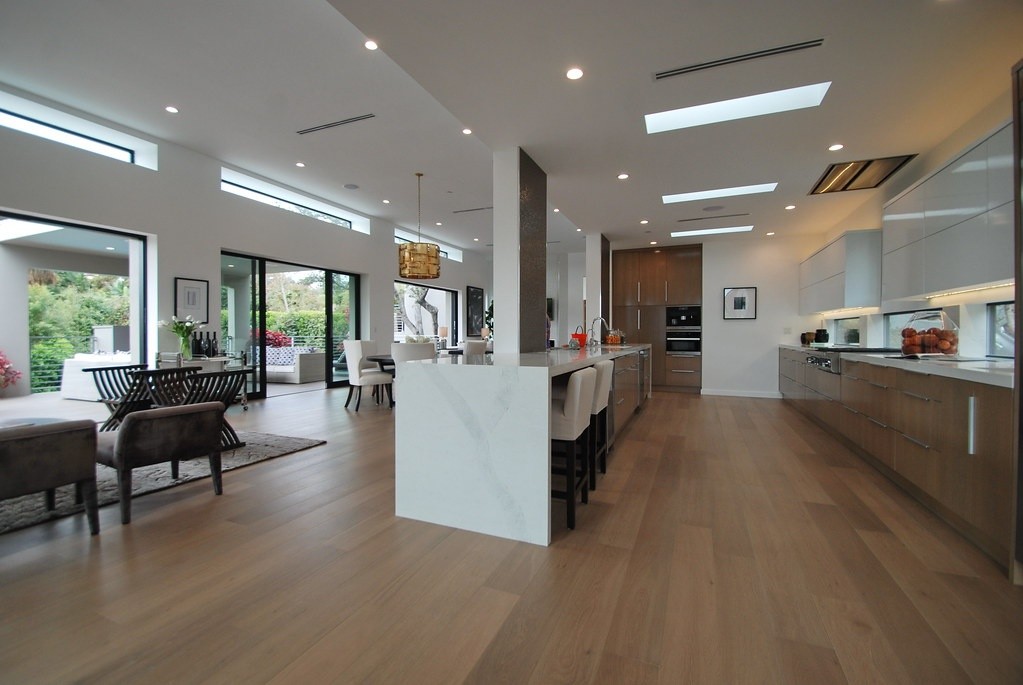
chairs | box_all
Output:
[463,340,487,355]
[343,340,436,412]
[0,419,100,535]
[72,401,226,524]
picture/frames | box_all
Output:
[466,285,484,337]
[174,277,209,324]
[723,287,757,320]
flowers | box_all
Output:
[158,315,207,359]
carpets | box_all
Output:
[0,430,328,535]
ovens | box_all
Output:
[665,305,701,355]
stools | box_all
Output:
[551,360,615,530]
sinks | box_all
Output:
[595,345,632,349]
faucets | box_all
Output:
[590,316,611,347]
[586,328,596,342]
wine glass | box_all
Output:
[213,340,219,356]
[201,339,209,357]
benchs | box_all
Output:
[246,346,326,385]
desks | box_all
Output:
[156,356,233,390]
[366,355,396,410]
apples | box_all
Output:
[902,328,958,355]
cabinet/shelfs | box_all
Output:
[609,347,651,444]
[612,244,702,393]
[778,348,1014,576]
[796,117,1014,322]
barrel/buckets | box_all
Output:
[572,326,587,347]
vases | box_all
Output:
[180,337,192,360]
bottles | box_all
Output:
[211,332,218,356]
[191,332,199,355]
[206,332,212,357]
[197,332,205,354]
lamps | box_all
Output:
[399,173,441,279]
